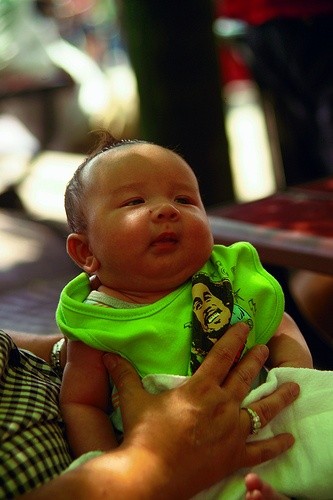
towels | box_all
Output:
[141,368,333,500]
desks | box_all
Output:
[204,175,333,276]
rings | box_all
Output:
[246,408,261,433]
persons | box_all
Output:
[0,273,333,500]
[63,141,333,500]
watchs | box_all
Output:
[51,338,64,374]
